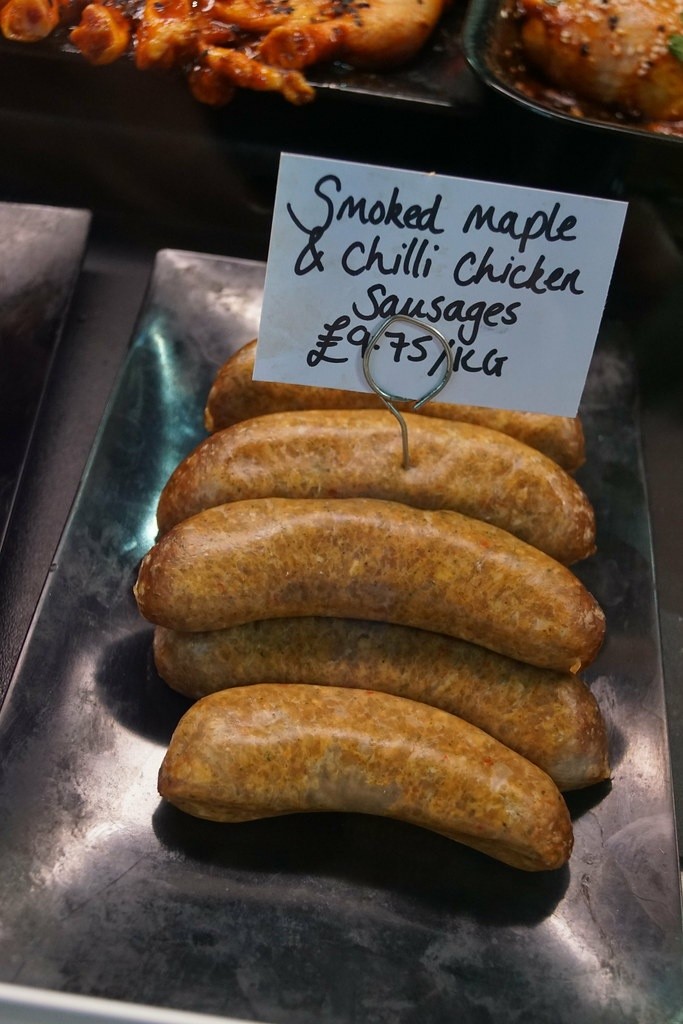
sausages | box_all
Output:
[134,337,612,873]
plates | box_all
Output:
[459,0,683,143]
[0,248,683,1024]
[0,203,93,549]
[59,20,483,109]
